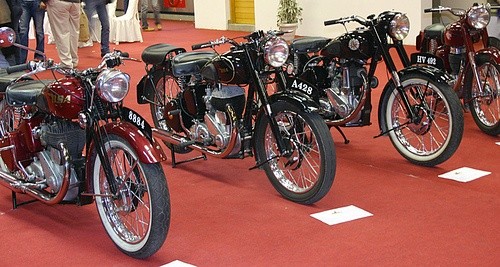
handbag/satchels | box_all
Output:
[78,7,90,42]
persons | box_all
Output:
[124,0,129,14]
[141,0,162,30]
[82,0,112,57]
[0,0,46,70]
[44,0,80,71]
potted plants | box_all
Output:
[276,0,303,42]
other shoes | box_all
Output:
[142,25,154,31]
[157,24,162,30]
[55,64,72,75]
[34,57,40,62]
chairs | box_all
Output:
[29,0,143,45]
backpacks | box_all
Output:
[0,0,13,24]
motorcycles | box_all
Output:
[273,11,465,167]
[415,2,500,136]
[0,26,172,260]
[136,28,337,205]
